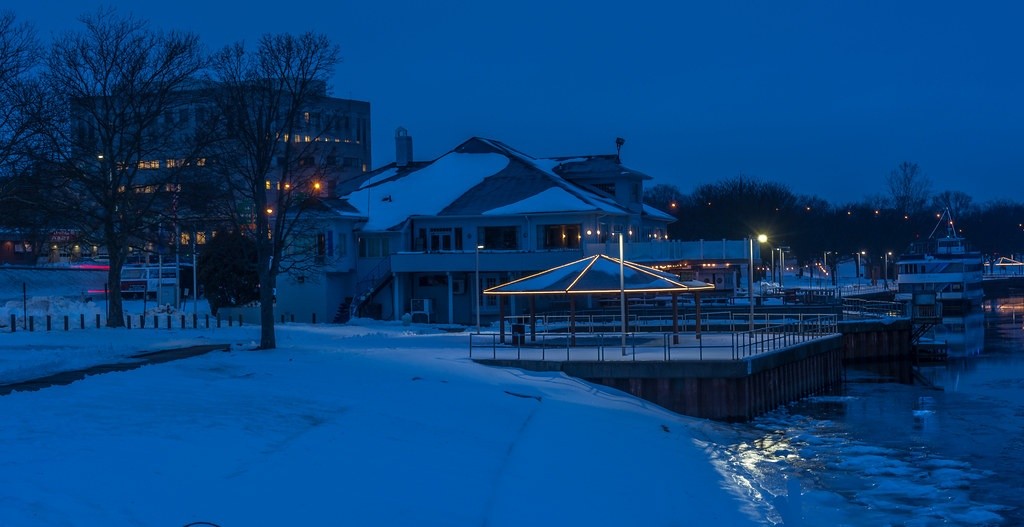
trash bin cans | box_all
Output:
[511,323,525,346]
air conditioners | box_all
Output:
[452,279,464,294]
[703,274,724,290]
[410,299,433,315]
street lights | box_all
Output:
[885,252,892,288]
[475,245,484,333]
[749,235,767,338]
[857,251,865,290]
[779,247,790,290]
[771,248,779,284]
